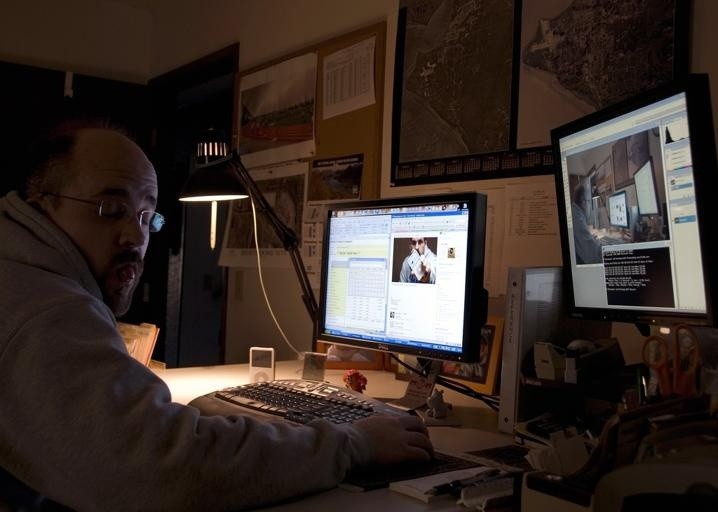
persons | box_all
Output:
[0,124,434,512]
[327,344,368,362]
[399,238,437,284]
[572,184,604,263]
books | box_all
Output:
[386,466,506,503]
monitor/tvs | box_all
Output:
[315,191,489,410]
[551,73,718,396]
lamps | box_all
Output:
[173,124,322,352]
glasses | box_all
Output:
[584,199,591,202]
[57,196,165,233]
[409,240,424,245]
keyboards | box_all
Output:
[187,378,408,425]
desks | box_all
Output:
[152,356,591,511]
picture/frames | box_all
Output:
[436,315,507,397]
[312,338,388,375]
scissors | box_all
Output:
[641,324,700,397]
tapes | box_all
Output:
[567,339,596,353]
